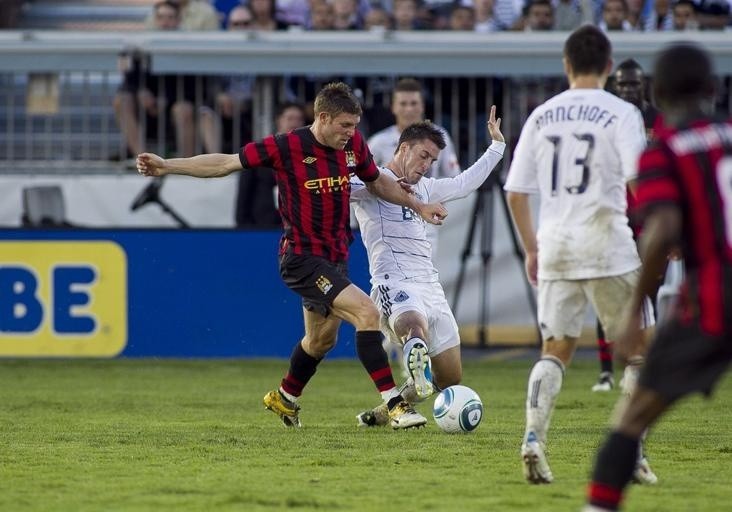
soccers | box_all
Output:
[434,384,483,434]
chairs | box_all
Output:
[19,184,73,226]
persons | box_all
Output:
[577,44,732,512]
[136,83,448,431]
[350,105,547,428]
[502,24,659,487]
[348,79,462,364]
[592,58,686,393]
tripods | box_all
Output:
[452,146,545,350]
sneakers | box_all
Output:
[520,439,556,485]
[263,388,304,428]
[387,402,427,432]
[590,369,617,391]
[357,404,393,426]
[631,459,659,485]
[407,343,436,397]
